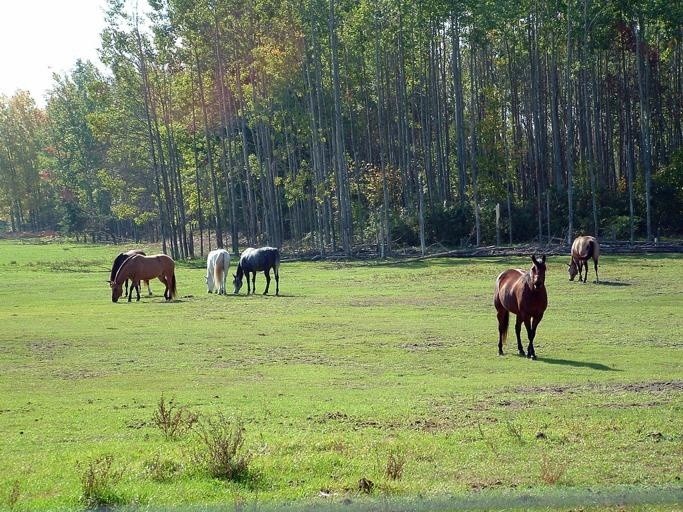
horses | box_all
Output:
[567,235,599,283]
[106,249,152,297]
[232,247,280,296]
[204,249,230,295]
[494,254,547,360]
[110,254,178,302]
